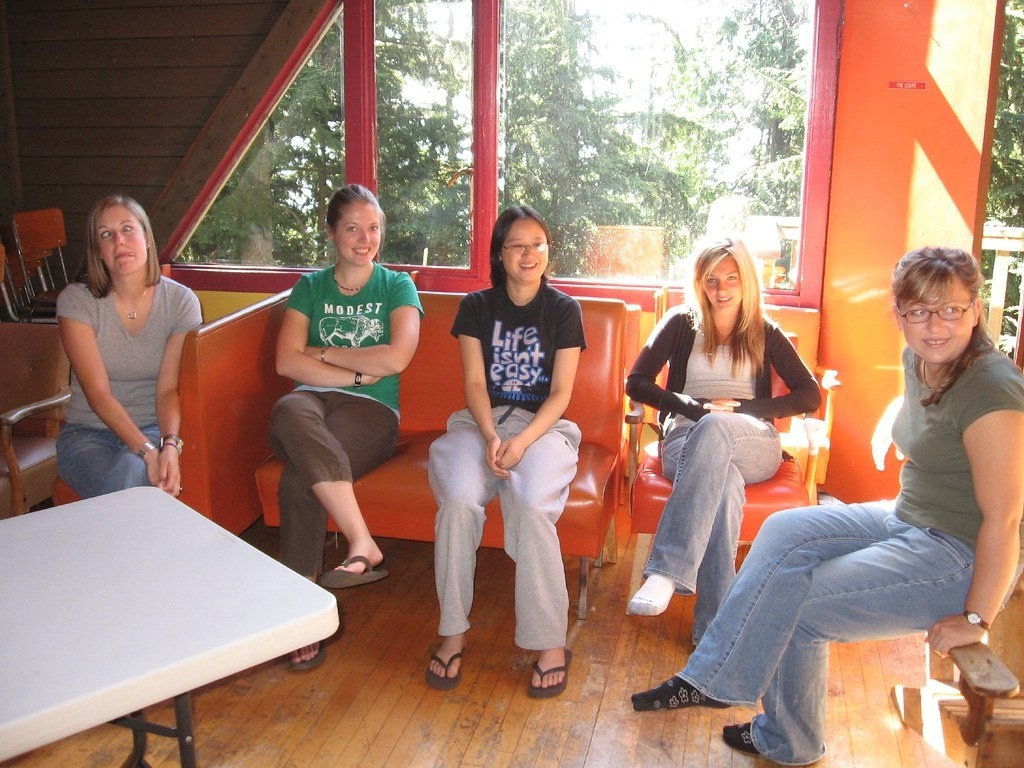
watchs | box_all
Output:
[963,610,991,631]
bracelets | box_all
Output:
[159,434,184,455]
[321,346,328,362]
[354,372,362,387]
[138,441,157,458]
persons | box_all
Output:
[55,193,203,500]
[426,206,587,697]
[631,248,1024,765]
[266,184,425,670]
[625,239,821,646]
[692,193,782,261]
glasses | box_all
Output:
[501,241,549,255]
[895,296,975,324]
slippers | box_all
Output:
[425,645,467,691]
[527,649,573,699]
[319,555,390,589]
[288,640,326,671]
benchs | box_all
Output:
[50,289,628,620]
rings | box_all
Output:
[178,486,182,491]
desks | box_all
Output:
[0,485,340,768]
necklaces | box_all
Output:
[924,361,937,389]
[334,266,362,291]
[113,287,147,318]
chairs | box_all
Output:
[890,623,1023,768]
[626,300,837,616]
[0,323,72,518]
[0,207,68,324]
